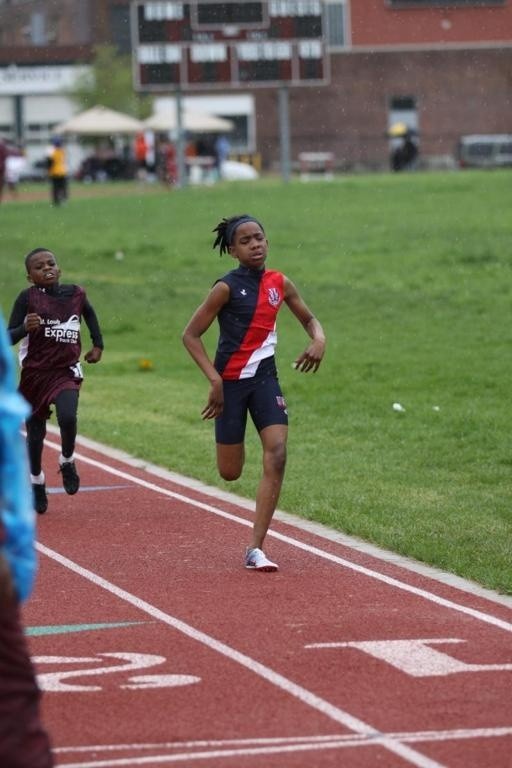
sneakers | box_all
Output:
[33,482,47,514]
[59,461,79,495]
[245,548,279,571]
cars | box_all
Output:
[74,153,156,184]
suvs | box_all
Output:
[456,132,512,168]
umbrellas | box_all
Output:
[139,103,238,137]
[53,104,146,141]
[386,123,417,136]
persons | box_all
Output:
[180,213,327,571]
[6,246,105,516]
[0,134,234,212]
[0,312,58,765]
[391,136,419,170]
